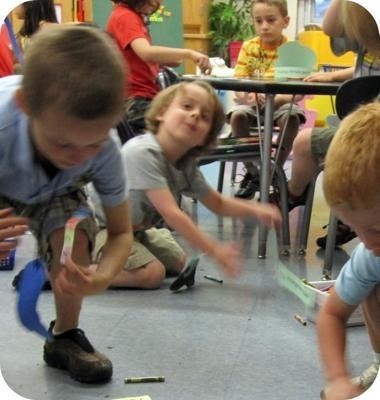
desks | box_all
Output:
[182,74,339,261]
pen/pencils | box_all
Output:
[294,313,306,326]
[124,376,165,383]
[203,275,223,283]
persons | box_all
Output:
[255,1,380,249]
[1,22,136,386]
[13,0,61,52]
[103,0,213,147]
[9,78,284,289]
[313,101,380,400]
[224,0,307,199]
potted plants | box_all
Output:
[203,0,259,72]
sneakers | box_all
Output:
[258,184,307,212]
[316,220,358,247]
[351,364,379,390]
[44,321,113,383]
[234,172,260,199]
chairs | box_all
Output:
[215,40,300,193]
[298,75,380,280]
[117,120,291,259]
